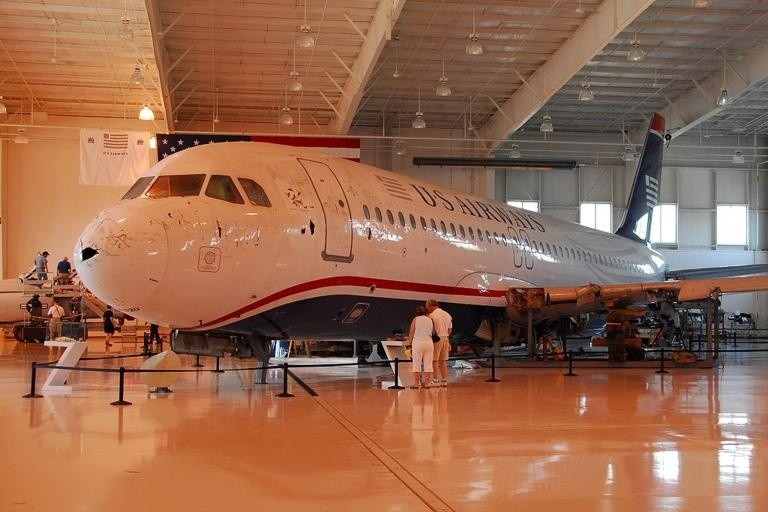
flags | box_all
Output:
[154,132,362,167]
[76,125,152,189]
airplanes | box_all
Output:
[74,111,768,358]
[0,252,136,339]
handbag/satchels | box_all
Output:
[431,318,441,343]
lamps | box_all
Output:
[716,46,732,109]
[730,127,747,165]
[392,0,484,155]
[276,1,315,126]
[13,92,31,145]
[119,1,155,123]
[508,29,644,160]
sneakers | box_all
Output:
[410,378,448,390]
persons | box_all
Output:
[26,293,43,343]
[148,324,161,344]
[426,299,453,387]
[47,300,65,341]
[34,251,49,280]
[103,304,115,346]
[406,305,435,389]
[57,256,72,284]
